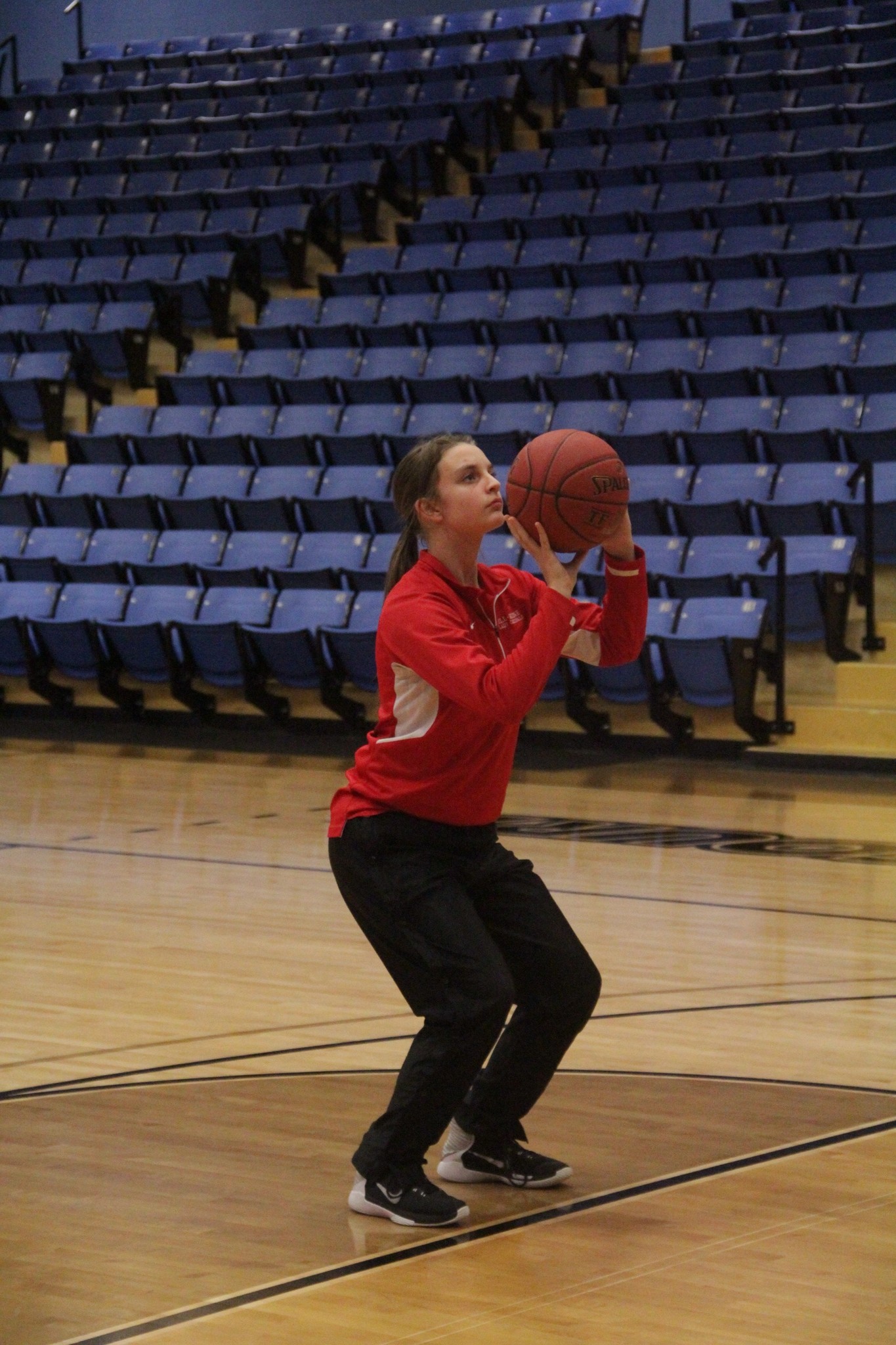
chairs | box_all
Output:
[0,0,895,748]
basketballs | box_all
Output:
[505,428,631,553]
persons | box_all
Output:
[326,433,649,1227]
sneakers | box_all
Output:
[346,1159,471,1228]
[436,1117,574,1191]
